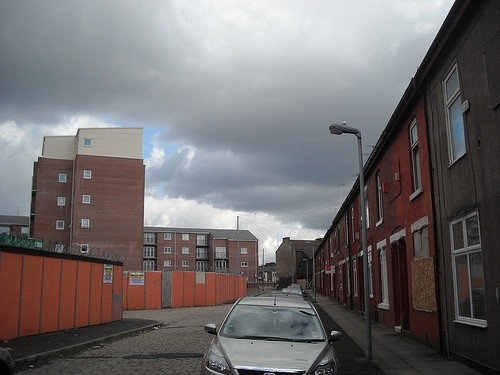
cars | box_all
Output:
[271,284,303,300]
[202,295,346,375]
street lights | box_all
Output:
[305,239,315,301]
[328,121,374,362]
[301,258,310,289]
